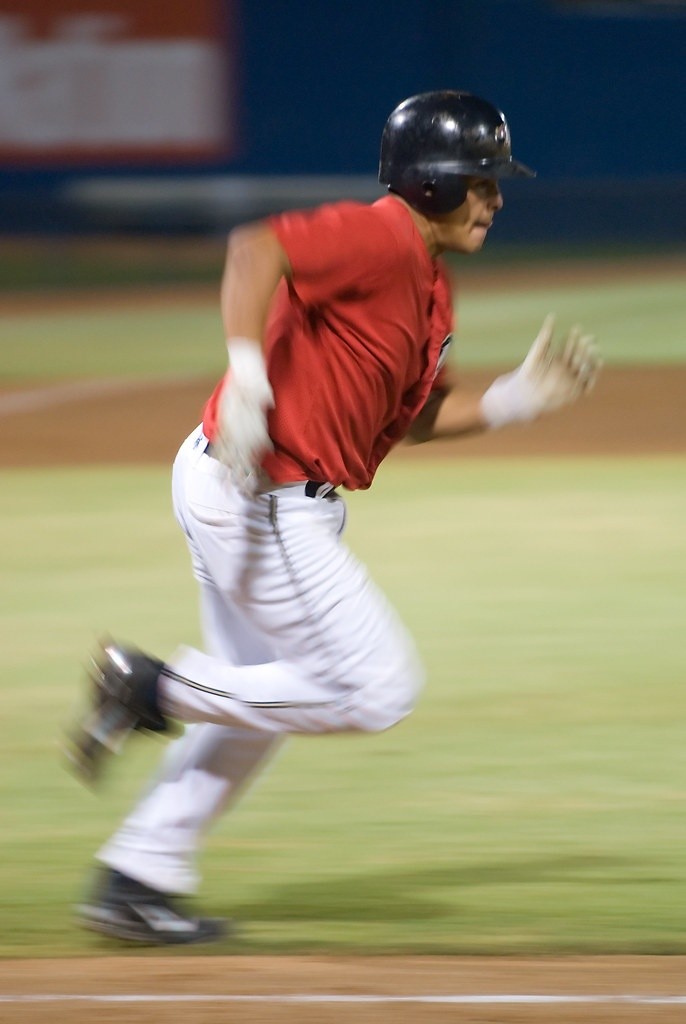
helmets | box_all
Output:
[378,92,537,217]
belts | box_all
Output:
[203,440,334,499]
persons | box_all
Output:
[61,92,599,939]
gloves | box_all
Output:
[215,337,275,496]
[481,316,605,430]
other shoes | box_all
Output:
[70,870,226,946]
[66,638,164,781]
